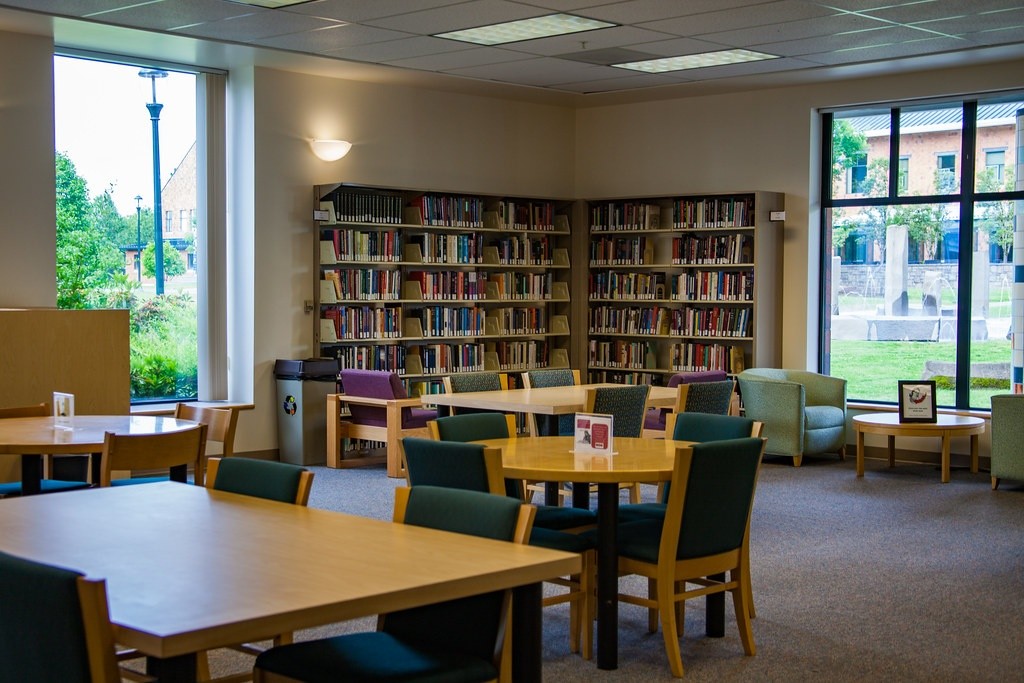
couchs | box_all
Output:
[737,366,848,466]
[990,394,1024,490]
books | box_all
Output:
[315,185,410,455]
[491,200,556,436]
[671,200,753,416]
[590,203,671,386]
[415,194,486,410]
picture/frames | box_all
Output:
[898,379,938,424]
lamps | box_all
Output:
[309,140,352,162]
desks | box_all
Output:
[0,475,582,683]
[461,431,703,671]
[0,412,201,496]
[852,409,985,483]
[419,380,679,437]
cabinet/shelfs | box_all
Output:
[312,184,578,404]
[578,191,785,384]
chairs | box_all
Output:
[0,367,767,683]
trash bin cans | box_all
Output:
[272,358,340,466]
